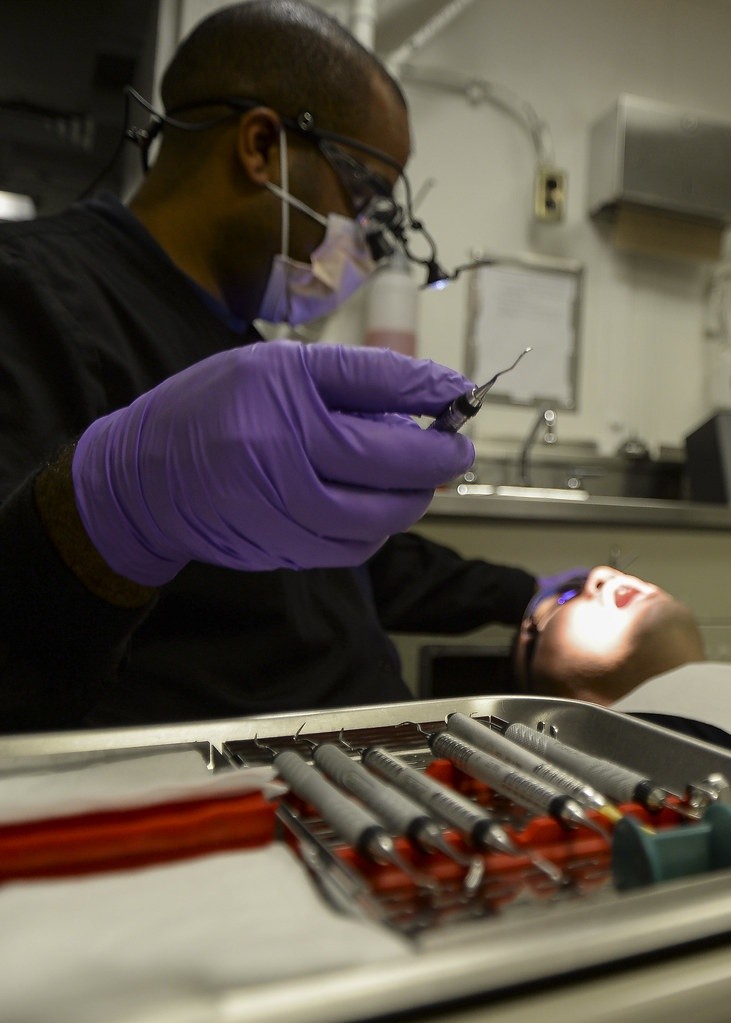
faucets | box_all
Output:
[519,404,558,484]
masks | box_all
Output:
[237,108,379,327]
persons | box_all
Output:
[509,567,730,749]
[0,1,549,736]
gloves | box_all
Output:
[72,339,477,588]
